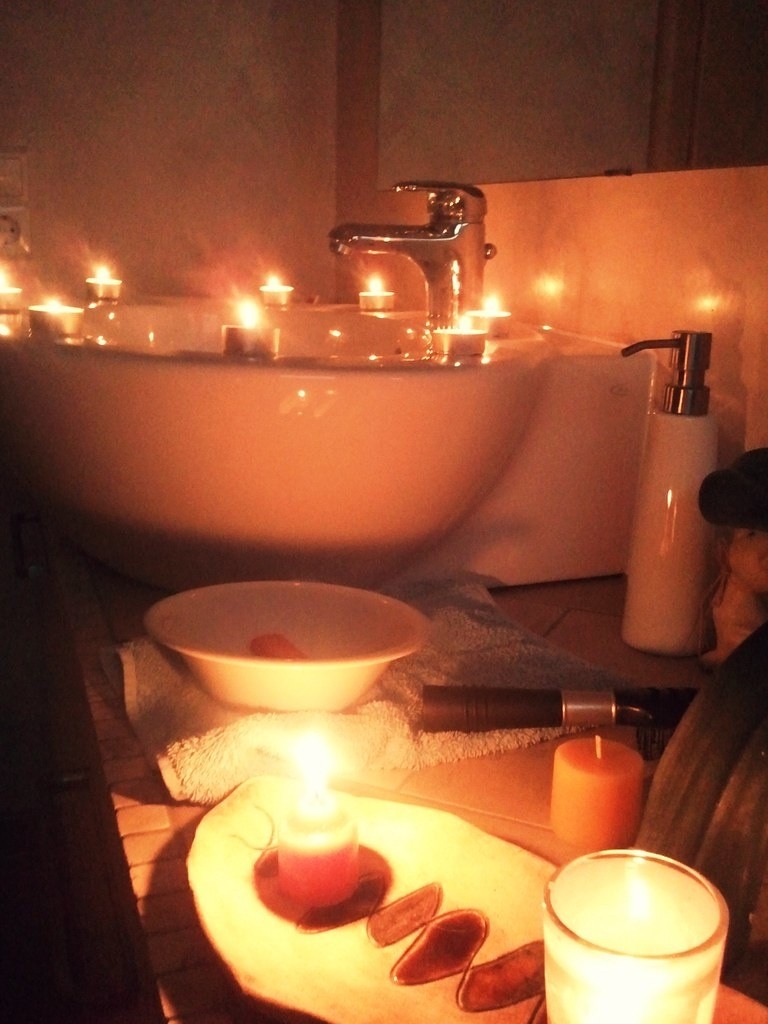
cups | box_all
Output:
[544,850,729,1024]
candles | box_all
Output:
[258,276,296,305]
[358,277,395,310]
[86,268,123,299]
[29,299,84,335]
[275,717,361,908]
[432,314,489,356]
[465,293,515,338]
[0,273,23,310]
[222,300,281,360]
[547,884,719,1024]
[548,735,645,857]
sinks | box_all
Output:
[0,292,657,592]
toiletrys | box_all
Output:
[617,330,721,658]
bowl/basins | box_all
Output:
[146,579,433,714]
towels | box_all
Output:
[97,579,597,809]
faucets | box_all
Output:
[327,176,491,334]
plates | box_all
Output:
[186,778,768,1024]
